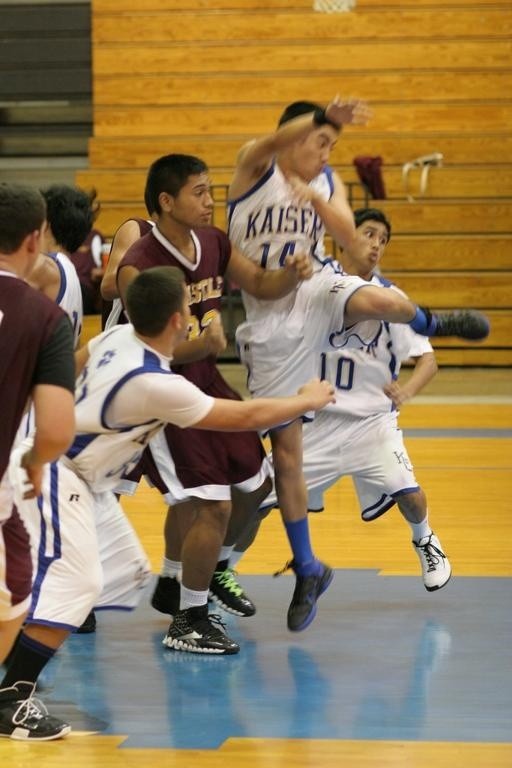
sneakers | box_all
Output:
[162,603,240,655]
[284,558,335,632]
[0,680,73,741]
[150,573,181,615]
[208,566,257,617]
[418,306,492,342]
[412,529,452,590]
